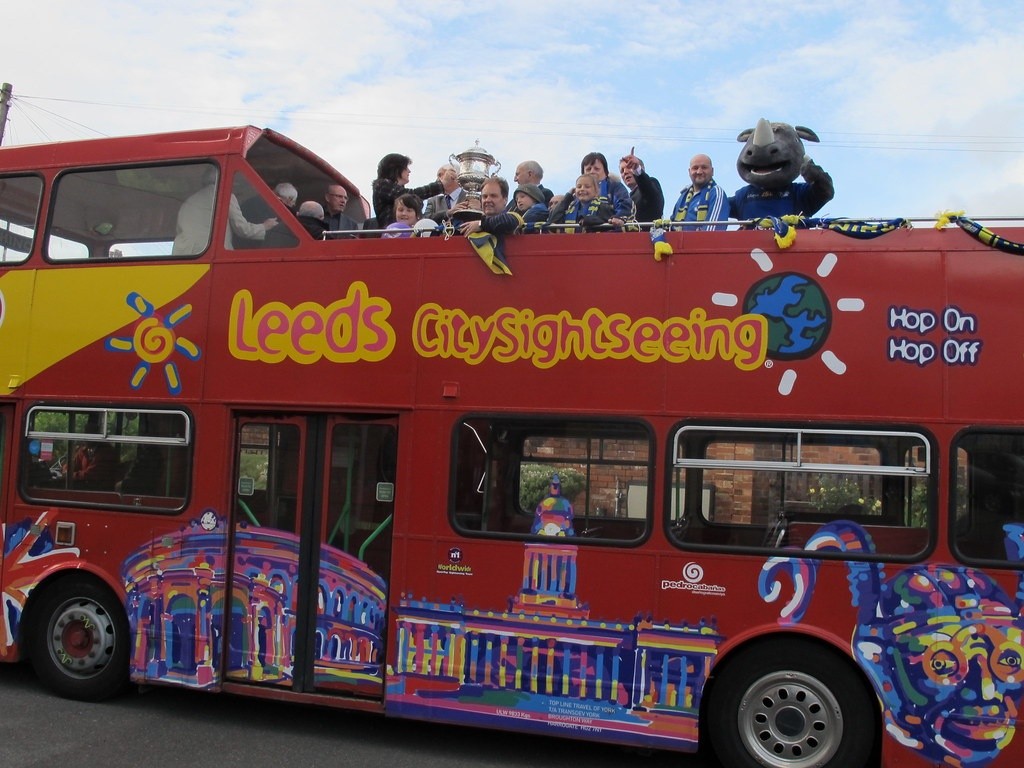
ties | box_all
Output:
[445,194,452,210]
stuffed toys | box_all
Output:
[727,117,835,221]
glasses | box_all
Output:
[329,194,349,202]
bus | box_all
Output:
[0,124,1024,768]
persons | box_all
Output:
[62,424,116,491]
[372,146,729,239]
[171,167,358,255]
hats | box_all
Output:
[513,183,545,203]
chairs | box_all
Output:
[126,455,165,495]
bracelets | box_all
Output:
[478,220,482,230]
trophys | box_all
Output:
[448,138,501,219]
